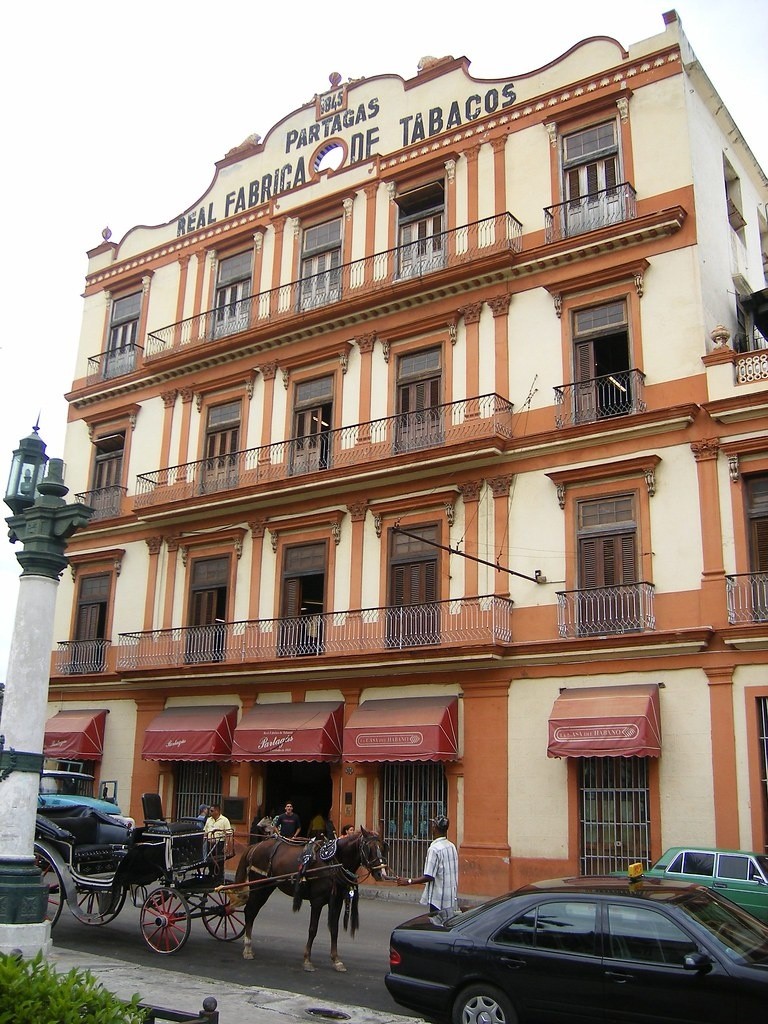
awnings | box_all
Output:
[42,709,108,762]
[546,684,661,758]
[142,695,457,763]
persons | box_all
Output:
[194,804,232,879]
[396,815,458,912]
[253,800,354,840]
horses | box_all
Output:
[234,825,390,973]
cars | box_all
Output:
[383,862,768,1024]
[607,846,768,924]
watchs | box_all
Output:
[407,878,411,884]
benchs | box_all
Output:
[47,817,128,875]
[143,791,208,872]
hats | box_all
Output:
[199,804,210,810]
[428,815,449,831]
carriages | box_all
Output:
[33,766,390,972]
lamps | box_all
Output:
[4,411,49,515]
